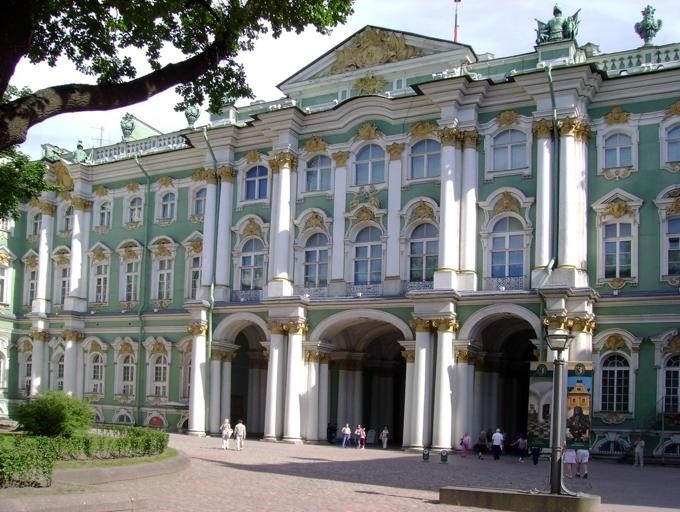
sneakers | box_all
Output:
[566,473,569,477]
[568,473,573,479]
[634,463,639,467]
[582,472,589,480]
[575,472,581,478]
[640,463,644,467]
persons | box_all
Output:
[354,424,363,449]
[381,426,389,448]
[332,423,339,443]
[633,435,645,467]
[460,428,542,466]
[233,419,246,451]
[327,423,334,444]
[341,423,351,448]
[220,418,231,450]
[575,449,590,479]
[563,449,577,479]
[360,427,366,448]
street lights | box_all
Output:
[541,314,573,494]
